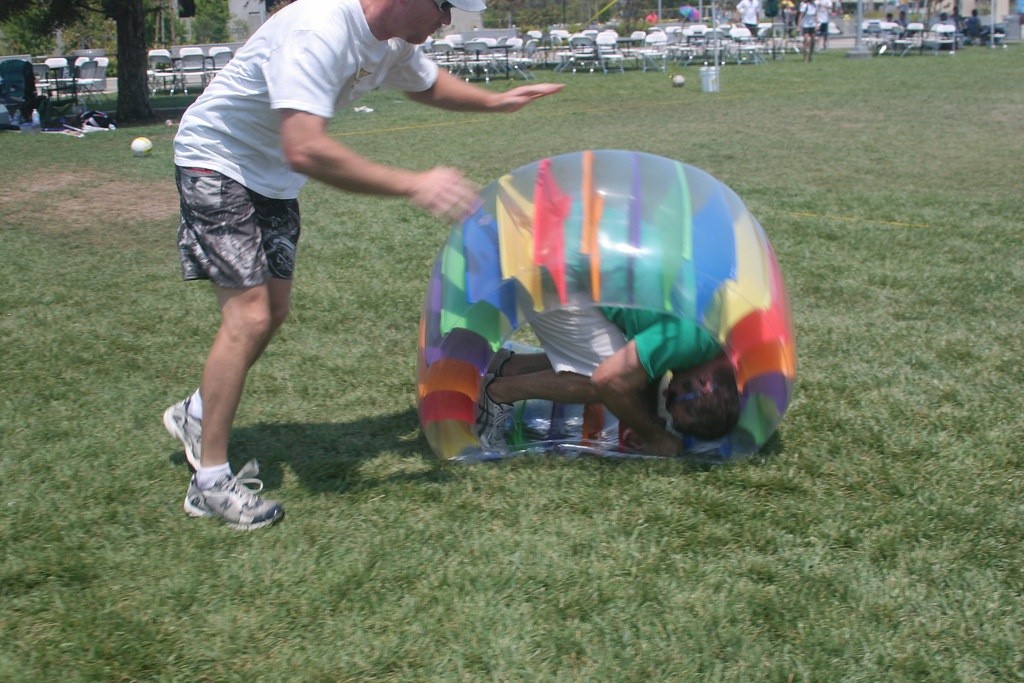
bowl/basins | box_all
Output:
[19,123,32,131]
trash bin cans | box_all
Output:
[0,58,34,120]
[702,65,718,92]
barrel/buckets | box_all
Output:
[700,66,720,93]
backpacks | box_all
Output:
[79,109,117,129]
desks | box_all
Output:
[169,55,215,92]
[688,34,705,43]
[617,36,641,56]
[445,44,471,74]
[476,45,514,79]
[45,65,82,98]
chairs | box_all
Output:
[524,23,819,74]
[31,57,110,107]
[422,35,535,84]
[147,46,233,97]
[861,15,1007,58]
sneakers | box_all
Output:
[183,459,282,530]
[163,388,202,470]
[470,373,514,455]
[486,348,514,377]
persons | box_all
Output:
[966,9,986,45]
[736,0,760,37]
[679,3,692,24]
[646,11,658,25]
[474,199,748,455]
[778,0,834,61]
[935,8,966,38]
[163,1,566,531]
[886,11,911,49]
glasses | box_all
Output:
[435,0,454,14]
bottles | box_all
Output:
[109,124,116,130]
[32,109,41,134]
[8,115,12,125]
[63,66,70,79]
[165,119,173,128]
[12,109,21,125]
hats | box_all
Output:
[447,0,487,12]
